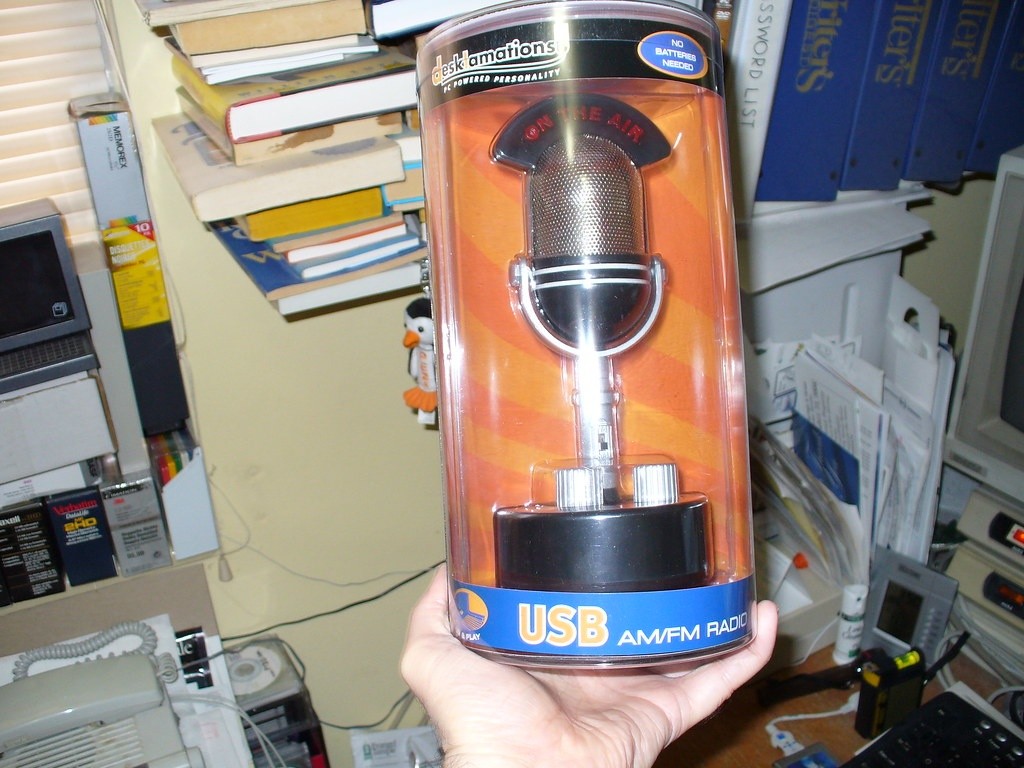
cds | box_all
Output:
[223,645,280,697]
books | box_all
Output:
[135,0,514,324]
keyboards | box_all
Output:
[840,692,1024,768]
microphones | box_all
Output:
[529,135,643,506]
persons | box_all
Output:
[398,560,778,768]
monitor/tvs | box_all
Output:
[0,197,92,354]
[940,145,1024,512]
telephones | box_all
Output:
[0,622,206,768]
